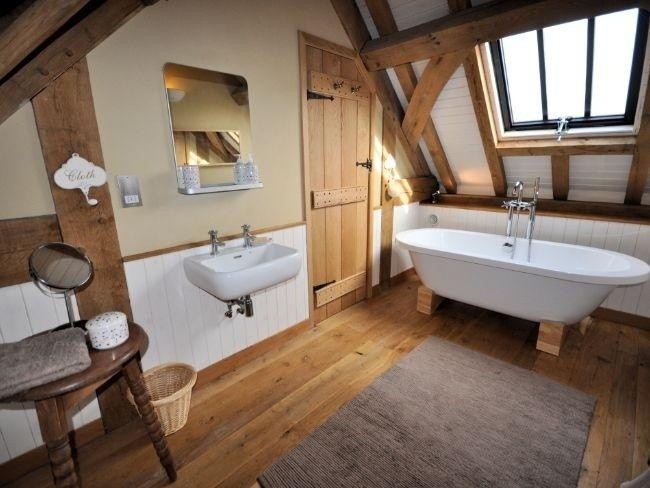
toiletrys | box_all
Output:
[232,153,244,184]
[244,152,259,184]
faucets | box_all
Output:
[240,223,256,249]
[512,179,524,239]
[208,230,225,257]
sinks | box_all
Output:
[183,241,301,302]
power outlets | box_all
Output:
[118,176,140,205]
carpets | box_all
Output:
[257,334,600,487]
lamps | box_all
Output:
[383,146,406,202]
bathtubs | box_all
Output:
[394,226,650,327]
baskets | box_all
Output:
[125,361,198,438]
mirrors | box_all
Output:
[160,64,263,194]
[33,246,96,322]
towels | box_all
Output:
[0,331,92,398]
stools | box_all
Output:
[7,315,180,488]
